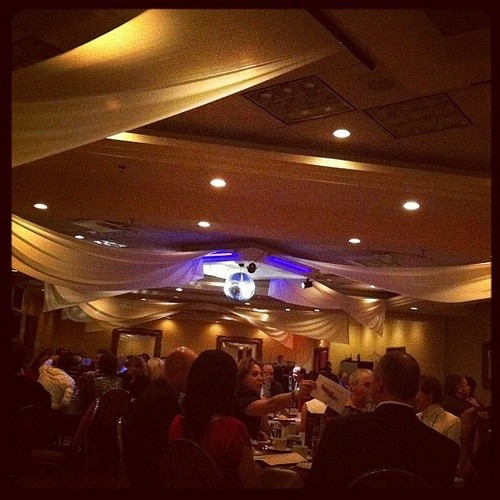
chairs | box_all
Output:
[10,387,227,489]
[346,465,433,489]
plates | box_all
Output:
[272,418,294,421]
[267,444,291,453]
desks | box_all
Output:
[249,432,315,489]
[268,407,304,435]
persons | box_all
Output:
[232,360,317,439]
[259,364,284,399]
[273,355,284,363]
[338,372,349,388]
[414,374,465,446]
[440,374,471,417]
[121,346,201,451]
[129,358,167,407]
[34,347,78,426]
[83,352,127,397]
[299,350,460,490]
[95,349,110,366]
[462,376,480,397]
[322,368,375,424]
[116,350,150,380]
[320,361,332,372]
[11,335,51,408]
[166,349,263,489]
[291,365,308,391]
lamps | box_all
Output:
[301,278,313,289]
[246,261,258,274]
[223,266,256,301]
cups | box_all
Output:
[251,442,259,452]
[259,441,270,451]
[291,444,313,459]
[272,436,287,449]
[287,433,300,447]
[268,407,301,420]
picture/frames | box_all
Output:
[481,340,493,388]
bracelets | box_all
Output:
[288,391,295,402]
